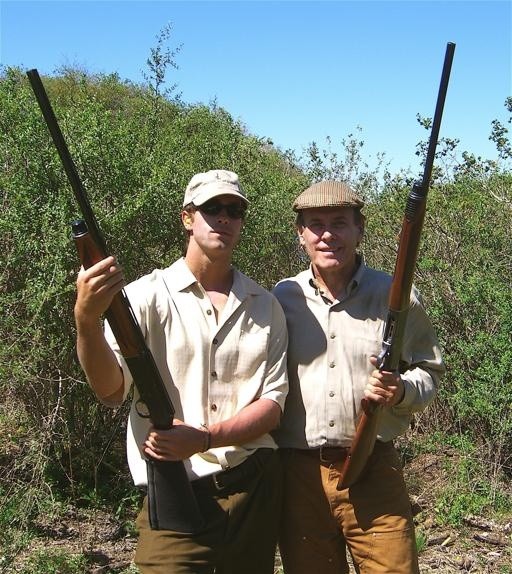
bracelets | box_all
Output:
[199,423,212,453]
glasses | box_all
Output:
[198,200,248,219]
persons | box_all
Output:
[268,179,446,574]
[71,166,291,574]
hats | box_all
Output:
[291,179,364,212]
[182,169,250,209]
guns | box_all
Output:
[335,41,455,484]
[26,68,207,533]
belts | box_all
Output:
[280,447,347,462]
[191,448,273,498]
